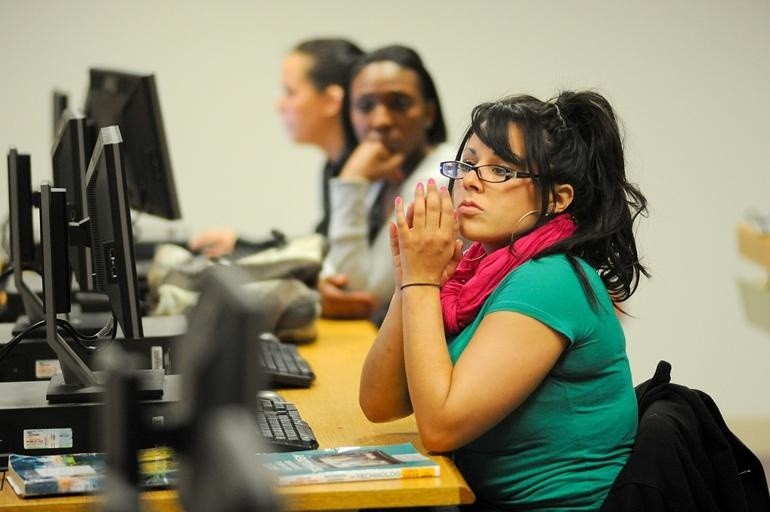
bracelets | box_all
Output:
[398,282,444,290]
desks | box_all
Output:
[0,235,481,512]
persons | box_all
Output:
[190,37,367,288]
[316,45,465,319]
[357,89,643,511]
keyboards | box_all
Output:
[247,338,314,388]
[250,397,320,450]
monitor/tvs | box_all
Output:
[6,66,182,404]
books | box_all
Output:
[257,442,442,487]
[9,450,181,498]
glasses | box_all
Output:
[439,161,538,184]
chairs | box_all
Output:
[602,363,770,511]
